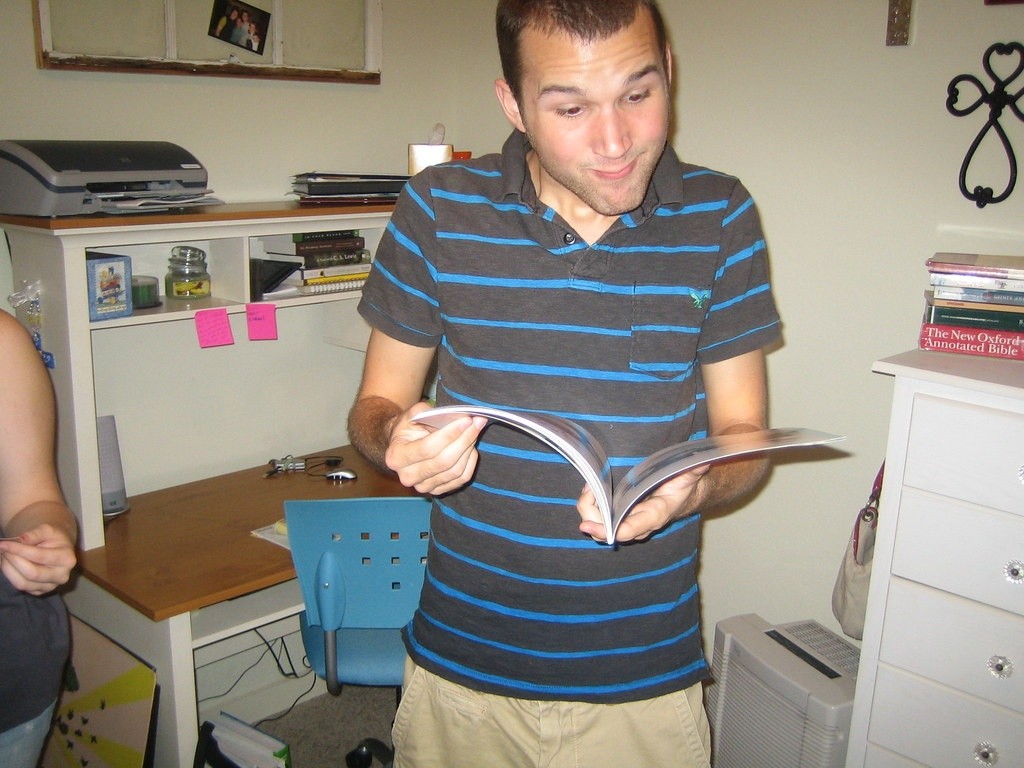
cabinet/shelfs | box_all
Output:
[0,198,392,551]
[844,348,1022,767]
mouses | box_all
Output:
[326,468,357,480]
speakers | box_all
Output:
[97,414,128,512]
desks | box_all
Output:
[58,442,429,768]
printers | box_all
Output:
[0,139,226,218]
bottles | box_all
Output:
[165,245,211,299]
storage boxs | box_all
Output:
[85,249,133,322]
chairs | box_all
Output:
[283,496,430,767]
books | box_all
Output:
[918,252,1024,360]
[208,711,291,768]
[250,230,372,302]
[289,172,414,203]
[412,405,848,545]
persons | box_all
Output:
[0,310,78,767]
[351,0,784,768]
[216,10,260,52]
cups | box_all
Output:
[131,275,159,307]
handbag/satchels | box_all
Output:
[831,462,887,641]
